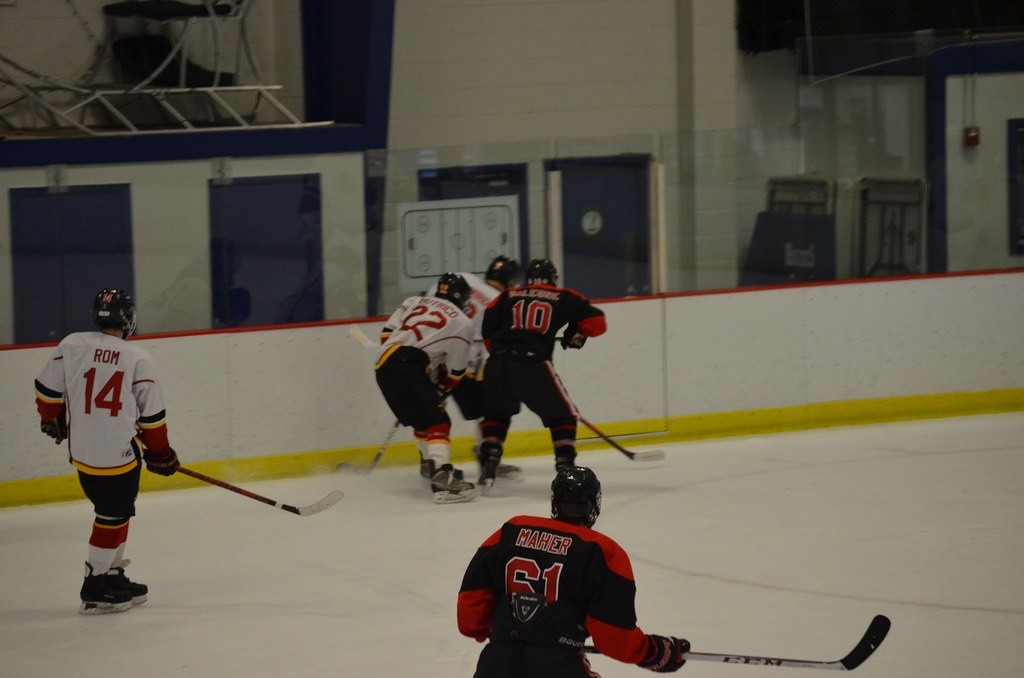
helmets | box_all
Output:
[435,272,471,310]
[484,255,521,285]
[93,289,137,340]
[549,467,601,526]
[526,259,557,285]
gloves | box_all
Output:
[143,447,180,476]
[40,412,67,445]
[561,327,586,350]
[637,634,690,672]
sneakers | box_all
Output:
[478,448,499,487]
[429,460,478,503]
[419,450,464,481]
[108,567,148,604]
[79,561,133,615]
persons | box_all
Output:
[374,271,477,504]
[426,254,521,479]
[457,467,691,678]
[478,258,608,495]
[36,289,180,617]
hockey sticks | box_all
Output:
[472,336,562,343]
[174,467,346,517]
[335,418,399,476]
[579,418,667,464]
[584,616,892,672]
[348,321,383,351]
